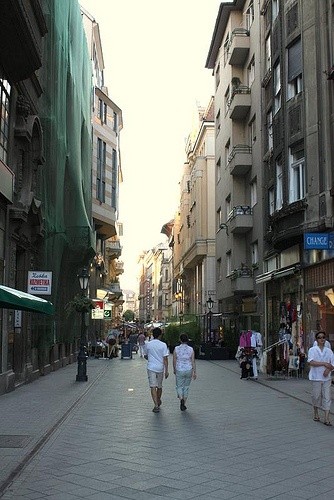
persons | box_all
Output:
[173,333,196,412]
[313,339,331,349]
[143,327,169,413]
[137,331,147,357]
[308,332,334,426]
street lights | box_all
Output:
[122,315,125,337]
[206,298,214,360]
[76,268,92,383]
[178,310,184,334]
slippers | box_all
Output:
[313,416,320,422]
[323,420,331,426]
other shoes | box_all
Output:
[180,399,187,411]
[155,400,161,406]
[153,407,160,412]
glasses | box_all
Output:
[317,336,326,339]
